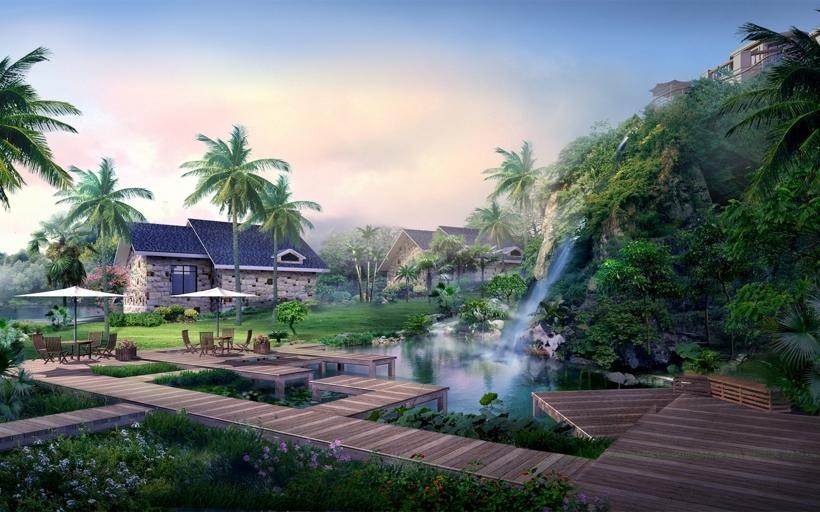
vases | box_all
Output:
[116,349,137,361]
[253,345,270,354]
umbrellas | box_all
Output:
[12,286,126,341]
[169,286,260,337]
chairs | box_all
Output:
[32,331,117,364]
[181,329,252,358]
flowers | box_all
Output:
[253,336,271,346]
[116,338,136,352]
